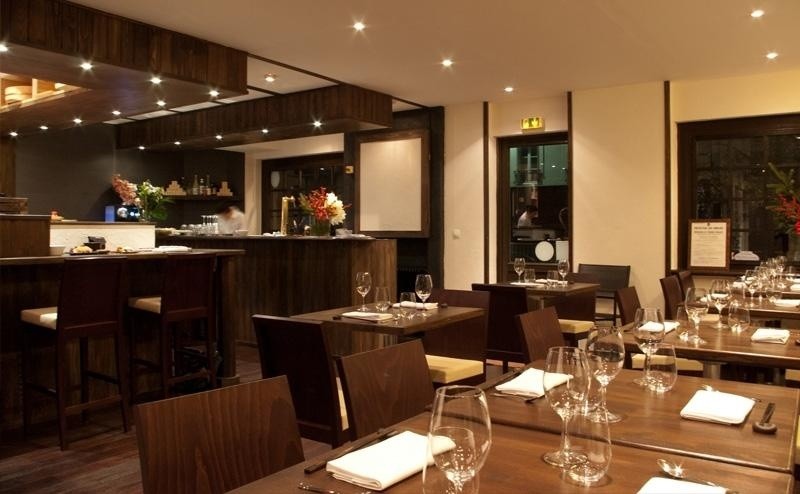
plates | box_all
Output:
[69,249,110,256]
[177,230,200,235]
[55,216,65,220]
[113,250,141,253]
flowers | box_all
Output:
[293,185,348,228]
[113,174,168,222]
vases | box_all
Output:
[138,208,156,223]
[308,217,331,236]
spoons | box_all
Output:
[490,391,543,401]
[655,458,738,493]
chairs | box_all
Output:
[517,307,568,365]
[615,286,645,330]
[472,281,528,364]
[424,290,495,395]
[254,315,349,449]
[660,275,683,321]
[135,373,304,491]
[577,262,631,328]
[129,251,221,401]
[332,340,435,435]
[677,270,697,299]
[17,255,127,449]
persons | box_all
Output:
[517,206,537,228]
[215,202,245,234]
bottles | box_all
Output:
[181,174,217,196]
[51,211,58,219]
[200,214,219,233]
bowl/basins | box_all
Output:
[83,242,102,250]
[236,229,249,236]
[50,245,66,255]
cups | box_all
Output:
[562,403,614,487]
[181,224,201,230]
[546,269,559,289]
[422,426,479,493]
[644,343,676,390]
[399,292,417,319]
[375,286,391,311]
[525,269,535,282]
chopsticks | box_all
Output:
[476,363,526,396]
[760,402,775,425]
[303,425,396,474]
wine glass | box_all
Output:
[633,306,665,386]
[559,260,570,287]
[513,257,525,284]
[415,273,434,318]
[542,346,590,468]
[430,385,493,493]
[587,323,625,422]
[354,272,373,312]
[676,255,796,344]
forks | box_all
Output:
[696,382,763,403]
